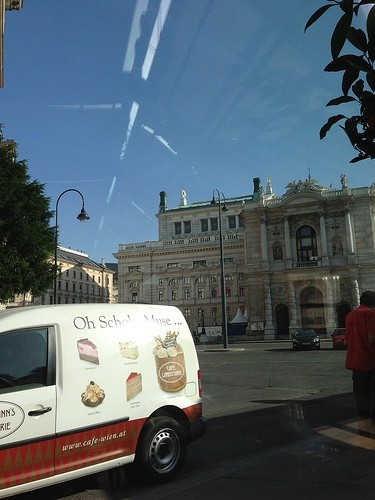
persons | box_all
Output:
[344,290,374,420]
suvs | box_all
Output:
[291,329,320,349]
[331,328,346,349]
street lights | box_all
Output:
[210,187,230,349]
[51,188,90,304]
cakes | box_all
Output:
[126,372,142,401]
[119,341,139,359]
[77,338,99,365]
[81,380,106,407]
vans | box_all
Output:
[1,304,203,496]
